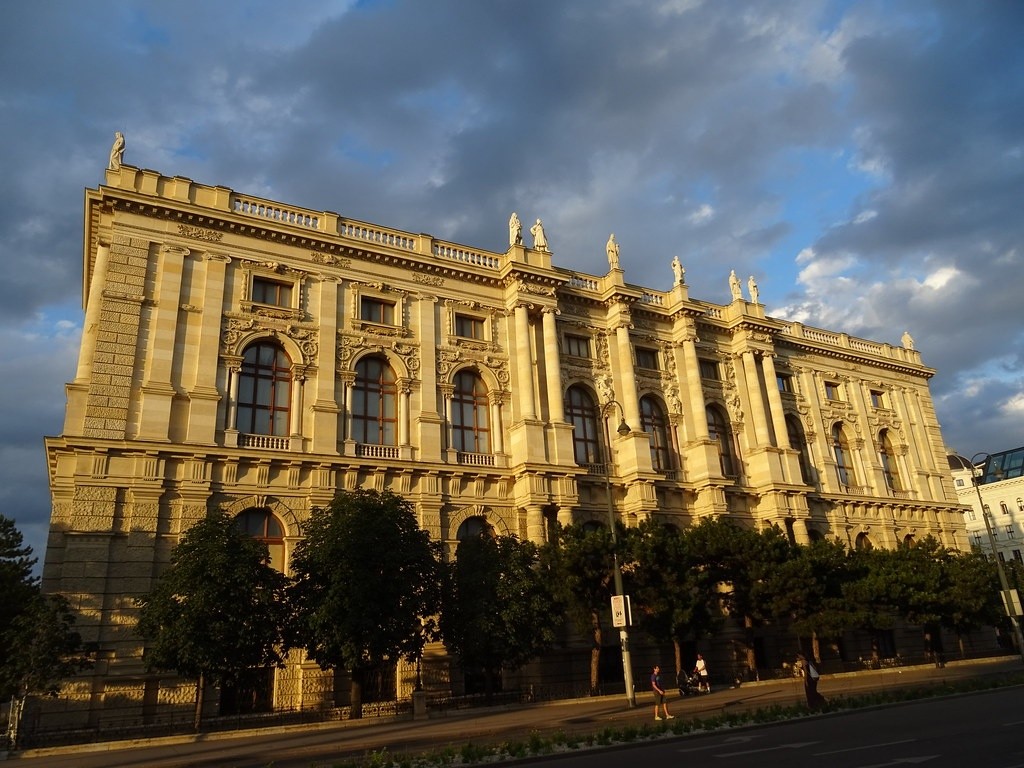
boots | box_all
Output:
[698,682,701,691]
[706,686,710,693]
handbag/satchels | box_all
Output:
[701,668,707,675]
[806,662,820,681]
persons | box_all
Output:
[924,633,945,668]
[797,650,825,711]
[694,652,710,695]
[109,132,124,171]
[729,270,744,300]
[509,213,523,245]
[531,219,551,253]
[901,332,914,349]
[748,276,759,302]
[672,256,686,283]
[607,234,619,270]
[651,665,674,720]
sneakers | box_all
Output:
[667,715,674,719]
[655,716,662,720]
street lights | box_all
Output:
[970,452,1024,662]
[600,400,636,710]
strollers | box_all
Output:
[677,669,699,697]
